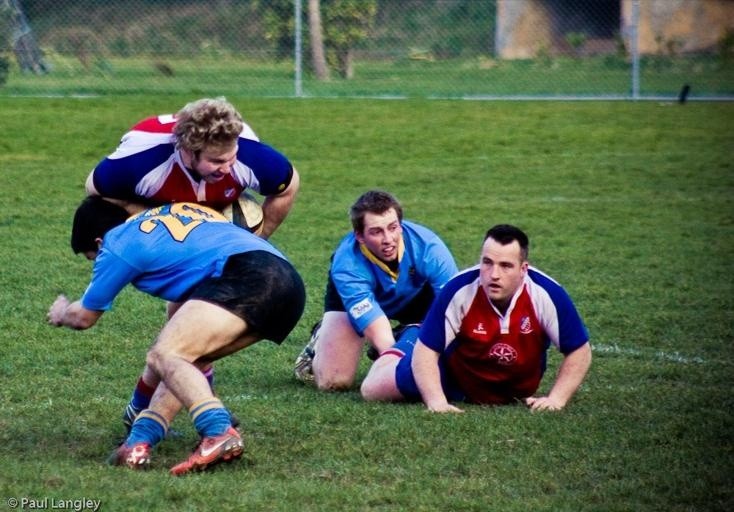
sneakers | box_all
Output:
[292,317,323,385]
[102,441,153,472]
[364,322,410,364]
[167,424,246,479]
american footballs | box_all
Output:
[223,192,264,236]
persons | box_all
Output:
[45,96,590,474]
